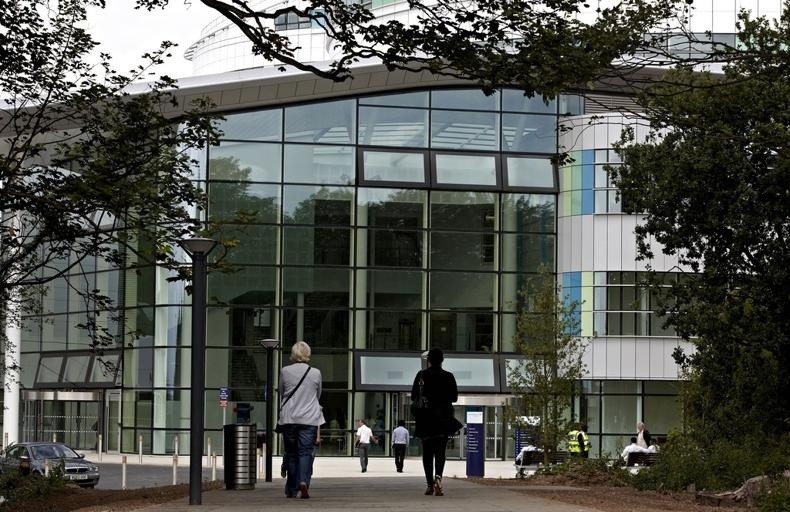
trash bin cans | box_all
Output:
[224,425,257,490]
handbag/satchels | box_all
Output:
[410,397,429,417]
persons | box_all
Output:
[515,438,544,474]
[567,422,593,458]
[619,421,663,466]
[409,346,463,496]
[390,420,409,472]
[354,419,379,472]
[275,341,325,499]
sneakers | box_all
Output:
[286,481,310,499]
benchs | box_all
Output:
[514,450,663,480]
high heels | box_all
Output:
[424,480,444,496]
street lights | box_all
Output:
[260,339,280,482]
[174,236,219,506]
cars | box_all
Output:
[0,441,102,490]
